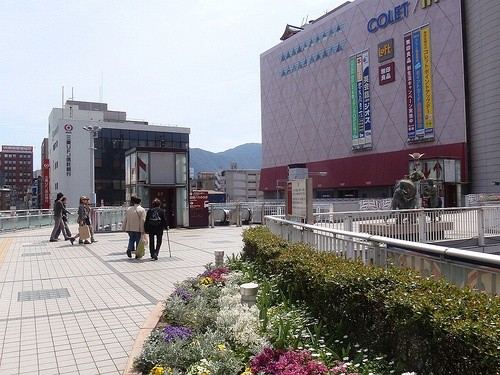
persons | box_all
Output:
[122,196,146,260]
[144,198,170,261]
[49,192,98,245]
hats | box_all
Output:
[80,196,88,200]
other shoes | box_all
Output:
[150,253,158,261]
[91,238,98,243]
[83,240,92,244]
[126,250,132,257]
[54,238,61,240]
[79,239,83,243]
[50,238,58,242]
[65,237,71,241]
[69,237,75,245]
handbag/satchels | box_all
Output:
[135,237,146,259]
